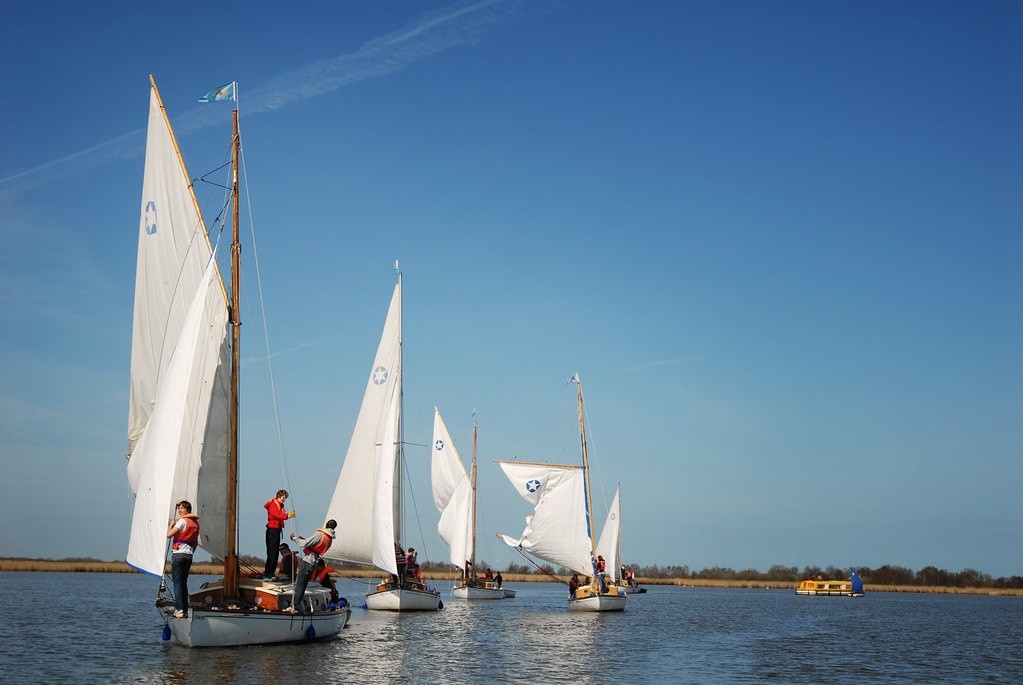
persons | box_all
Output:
[392,541,422,588]
[485,568,493,588]
[621,563,633,585]
[493,571,503,589]
[167,501,200,618]
[278,519,339,613]
[595,555,606,592]
[579,576,590,587]
[263,489,296,579]
[569,573,580,598]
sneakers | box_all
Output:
[172,609,187,618]
[282,607,299,613]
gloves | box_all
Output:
[290,533,294,540]
[415,552,417,556]
[298,536,305,540]
[288,511,296,519]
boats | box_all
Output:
[795,574,867,598]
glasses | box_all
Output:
[279,548,286,550]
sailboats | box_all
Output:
[596,482,649,595]
[118,75,362,652]
[495,372,629,612]
[318,259,453,617]
[431,407,519,601]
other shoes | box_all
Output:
[264,574,279,581]
